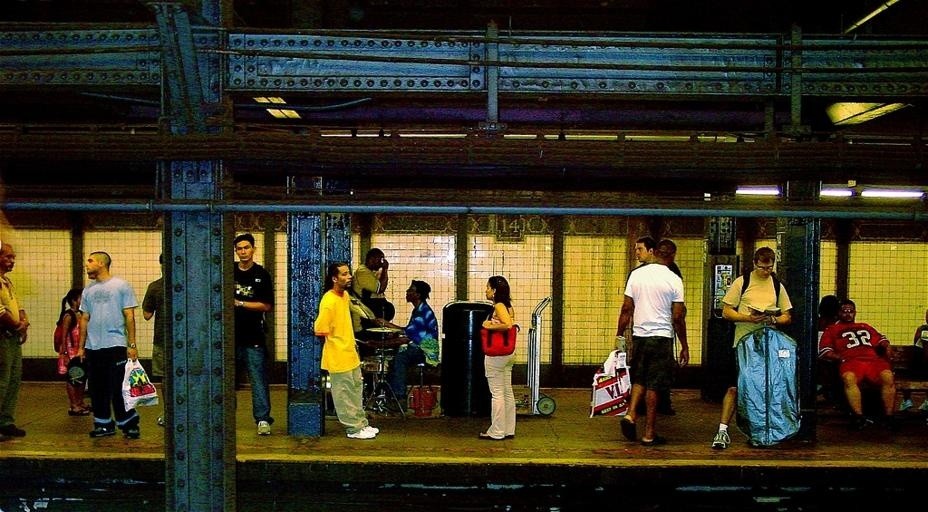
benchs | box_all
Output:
[817,345,928,443]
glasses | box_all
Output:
[840,309,855,313]
[756,264,774,271]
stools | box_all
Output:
[404,361,438,416]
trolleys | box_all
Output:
[512,296,557,417]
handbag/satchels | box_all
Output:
[481,325,517,357]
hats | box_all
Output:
[412,280,431,299]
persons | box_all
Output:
[142,253,167,427]
[899,324,924,412]
[627,236,681,416]
[313,262,380,438]
[819,301,898,435]
[480,276,516,440]
[712,246,793,451]
[818,295,840,329]
[235,233,273,437]
[1,243,30,441]
[55,288,91,416]
[77,253,142,441]
[353,248,389,301]
[377,281,438,411]
[617,240,686,445]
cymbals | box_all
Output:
[366,328,401,333]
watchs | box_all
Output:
[238,301,244,307]
[127,343,137,348]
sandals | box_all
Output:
[67,398,90,415]
[621,415,666,446]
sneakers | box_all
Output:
[713,430,730,448]
[1,424,25,437]
[347,425,380,438]
[90,426,115,438]
[479,431,494,440]
[257,421,271,436]
[123,426,139,440]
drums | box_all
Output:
[405,386,442,418]
[363,356,392,373]
